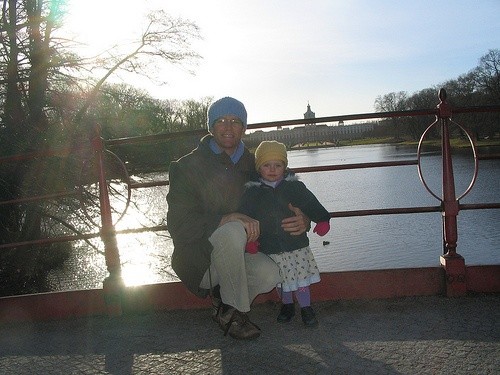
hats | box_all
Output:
[255,140,288,171]
[206,96,247,135]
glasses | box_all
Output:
[216,118,242,126]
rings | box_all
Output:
[251,232,255,234]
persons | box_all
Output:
[240,140,331,328]
[166,97,311,340]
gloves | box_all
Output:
[313,221,331,236]
[244,240,260,254]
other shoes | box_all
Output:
[301,305,318,324]
[277,303,295,322]
[211,299,221,323]
[217,304,261,340]
